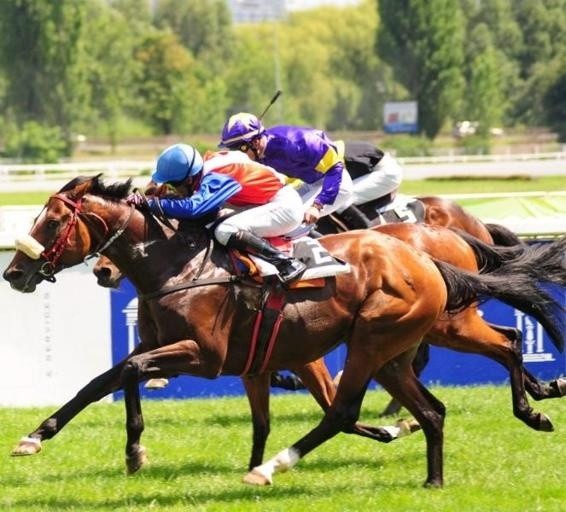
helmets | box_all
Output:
[152,143,203,182]
[218,113,265,147]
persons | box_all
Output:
[218,112,356,243]
[119,142,306,289]
[329,140,403,227]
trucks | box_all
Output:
[381,99,420,136]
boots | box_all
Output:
[225,230,306,288]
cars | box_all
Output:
[454,121,504,140]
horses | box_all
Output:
[1,172,566,491]
